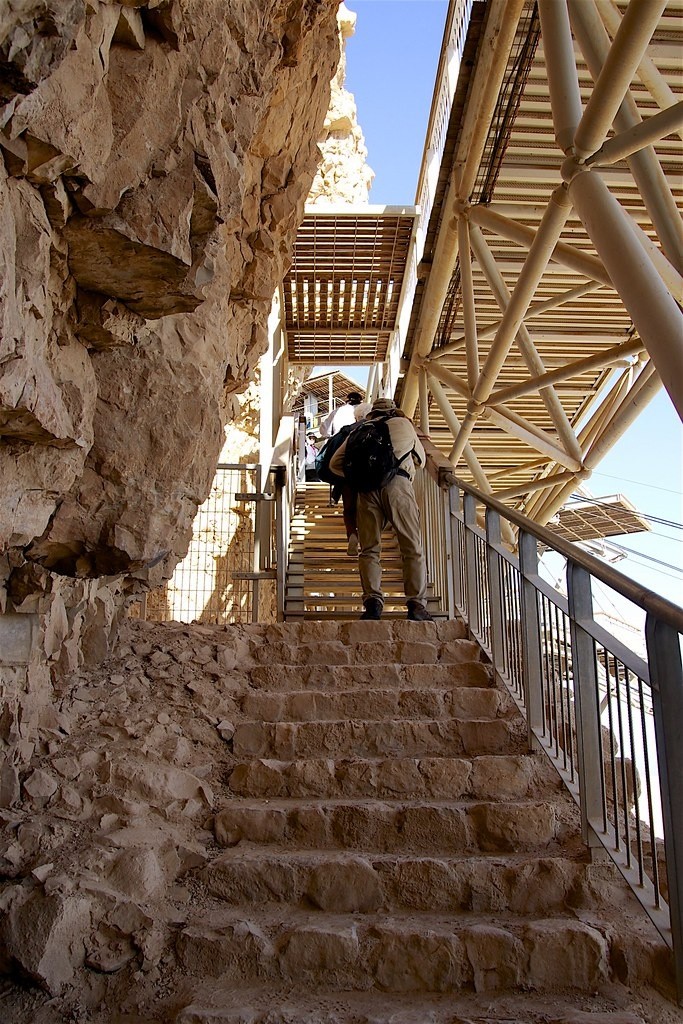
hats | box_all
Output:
[366,397,405,420]
[348,392,362,401]
[307,432,317,440]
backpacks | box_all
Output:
[344,416,414,492]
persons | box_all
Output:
[329,398,435,622]
[319,393,364,509]
[304,431,320,482]
[329,402,394,555]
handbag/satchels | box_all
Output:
[318,423,355,485]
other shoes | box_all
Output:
[331,491,338,506]
[348,533,359,556]
[404,600,432,620]
[383,519,392,531]
[359,597,383,620]
